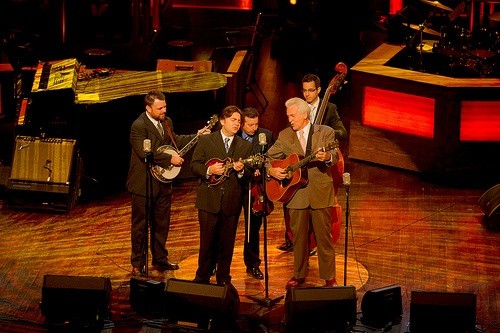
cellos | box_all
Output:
[283,61,348,252]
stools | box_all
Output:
[169,39,194,56]
[85,48,112,65]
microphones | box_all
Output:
[143,139,151,152]
[259,133,267,145]
[343,172,352,186]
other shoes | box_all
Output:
[286,277,305,289]
[325,279,338,287]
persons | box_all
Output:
[190,106,254,295]
[237,109,274,280]
[277,74,348,251]
[265,97,337,290]
[128,91,211,277]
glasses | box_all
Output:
[301,88,317,93]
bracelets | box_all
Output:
[326,154,333,164]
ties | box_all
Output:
[224,137,230,153]
[156,122,164,137]
[298,130,306,154]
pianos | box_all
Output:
[14,57,228,155]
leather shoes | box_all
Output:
[246,266,264,280]
[132,266,146,277]
[276,240,294,251]
[152,259,180,271]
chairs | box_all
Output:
[216,13,268,111]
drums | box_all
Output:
[409,25,500,78]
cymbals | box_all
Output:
[402,23,441,37]
[421,0,453,12]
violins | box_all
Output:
[251,168,274,216]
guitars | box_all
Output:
[265,139,341,203]
[148,114,219,184]
[203,152,263,186]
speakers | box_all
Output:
[287,287,356,333]
[164,279,240,323]
[361,283,403,325]
[410,292,477,333]
[40,274,112,322]
